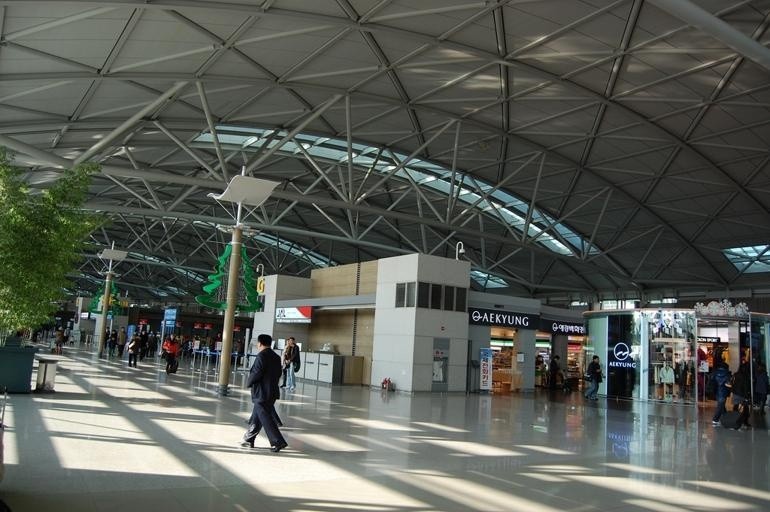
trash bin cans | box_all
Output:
[35,359,58,393]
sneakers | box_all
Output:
[585,392,598,400]
[280,384,296,390]
[743,422,751,427]
[713,418,721,425]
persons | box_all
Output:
[712,348,770,432]
[280,338,290,388]
[239,333,288,452]
[550,354,561,400]
[283,336,301,390]
[536,355,546,387]
[105,321,179,376]
[50,326,75,352]
[584,355,606,401]
[236,338,243,353]
[188,332,201,357]
[244,403,283,427]
[213,333,222,351]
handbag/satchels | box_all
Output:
[721,381,735,392]
[584,373,592,382]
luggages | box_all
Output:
[720,402,748,429]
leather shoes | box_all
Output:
[272,442,287,452]
[241,442,254,447]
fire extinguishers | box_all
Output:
[382,377,391,390]
